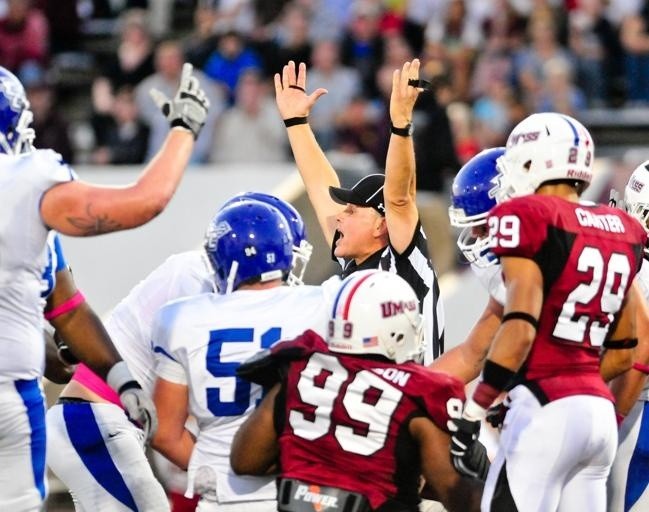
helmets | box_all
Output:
[625,159,649,235]
[0,66,37,154]
[202,201,294,294]
[328,268,426,365]
[221,192,313,286]
[448,146,505,268]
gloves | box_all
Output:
[150,63,210,140]
[450,416,486,477]
[117,380,157,438]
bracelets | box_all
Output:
[409,76,431,94]
[282,115,309,127]
[499,312,538,332]
[286,82,304,92]
[389,120,416,137]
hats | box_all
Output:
[497,112,594,199]
[329,173,385,217]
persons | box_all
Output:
[0,61,214,512]
[147,199,349,511]
[269,52,452,372]
[0,0,649,192]
[447,110,646,511]
